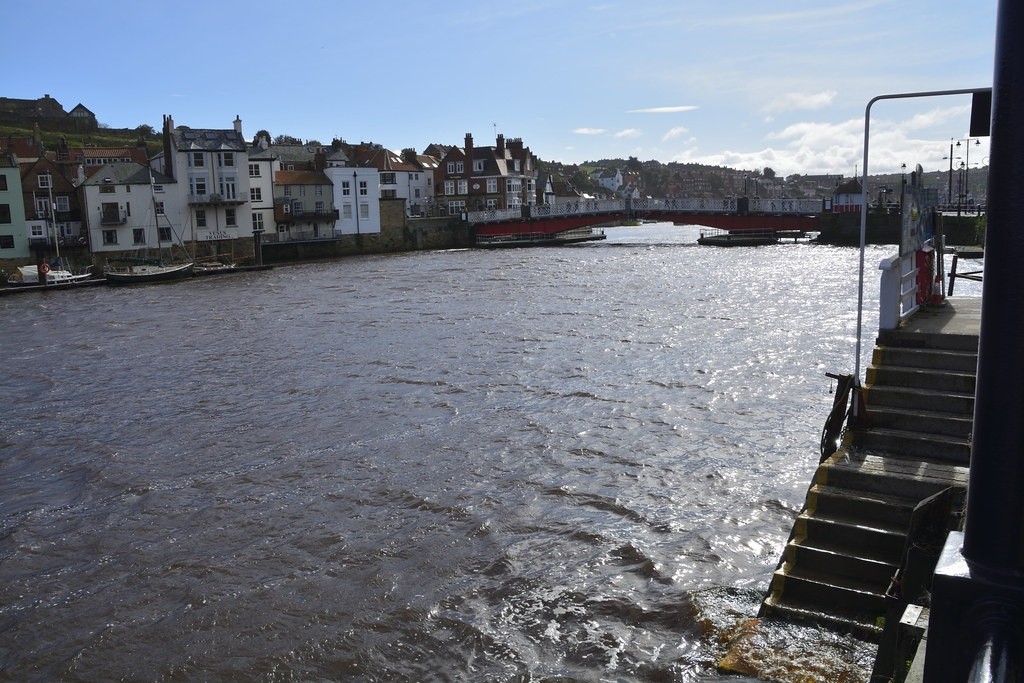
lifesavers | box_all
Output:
[39,263,50,274]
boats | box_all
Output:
[193,261,237,274]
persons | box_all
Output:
[587,201,592,211]
[789,201,793,211]
[782,196,786,210]
[544,200,550,213]
[664,196,671,210]
[672,197,678,209]
[574,201,579,212]
[594,202,599,211]
[566,200,571,213]
[969,196,974,213]
[700,197,705,209]
[887,199,892,207]
[754,194,760,210]
[770,195,776,210]
[723,195,735,210]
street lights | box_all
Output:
[960,161,965,207]
[898,163,907,256]
[942,157,961,203]
[744,174,748,196]
[754,176,758,196]
[955,138,981,214]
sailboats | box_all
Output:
[0,168,93,288]
[101,166,195,284]
